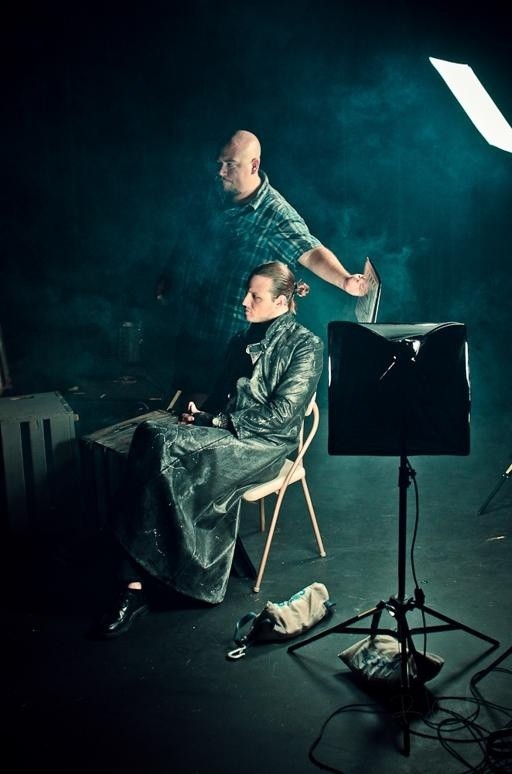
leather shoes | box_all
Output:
[105,592,149,637]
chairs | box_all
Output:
[240,396,329,595]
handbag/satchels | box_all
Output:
[233,582,336,648]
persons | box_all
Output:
[152,131,371,417]
[99,260,325,640]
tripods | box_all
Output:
[288,456,500,756]
